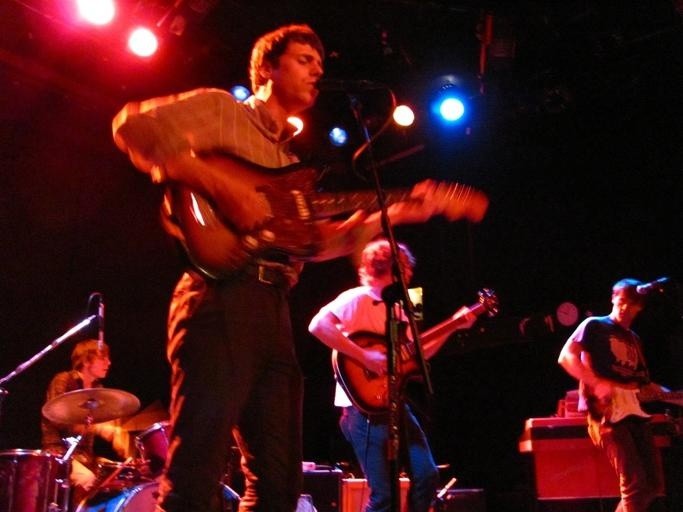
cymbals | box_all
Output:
[42,387,142,425]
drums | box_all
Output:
[95,461,144,492]
[0,448,73,511]
[85,478,237,511]
[135,420,175,476]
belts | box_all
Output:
[242,261,289,285]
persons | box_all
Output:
[306,238,478,511]
[113,23,467,511]
[556,277,682,511]
[39,339,151,512]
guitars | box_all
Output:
[331,287,500,417]
[165,148,491,286]
[587,377,683,427]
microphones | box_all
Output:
[95,301,109,357]
[313,72,377,93]
[635,276,668,295]
